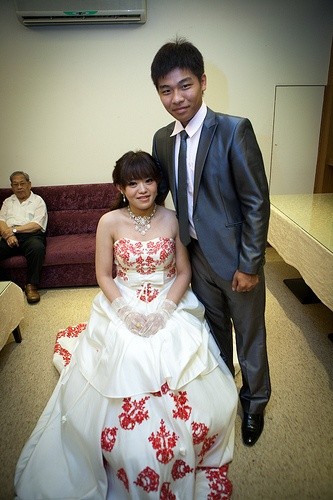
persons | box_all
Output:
[18,150,238,500]
[0,171,47,304]
[117,36,272,446]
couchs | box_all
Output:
[0,184,121,290]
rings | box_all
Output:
[136,323,140,327]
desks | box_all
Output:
[268,193,333,343]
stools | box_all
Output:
[0,280,27,351]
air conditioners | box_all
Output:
[15,0,147,28]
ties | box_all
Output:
[178,130,191,247]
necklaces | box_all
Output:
[128,202,157,235]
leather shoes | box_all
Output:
[241,412,264,446]
[25,284,40,303]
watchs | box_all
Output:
[12,226,17,234]
